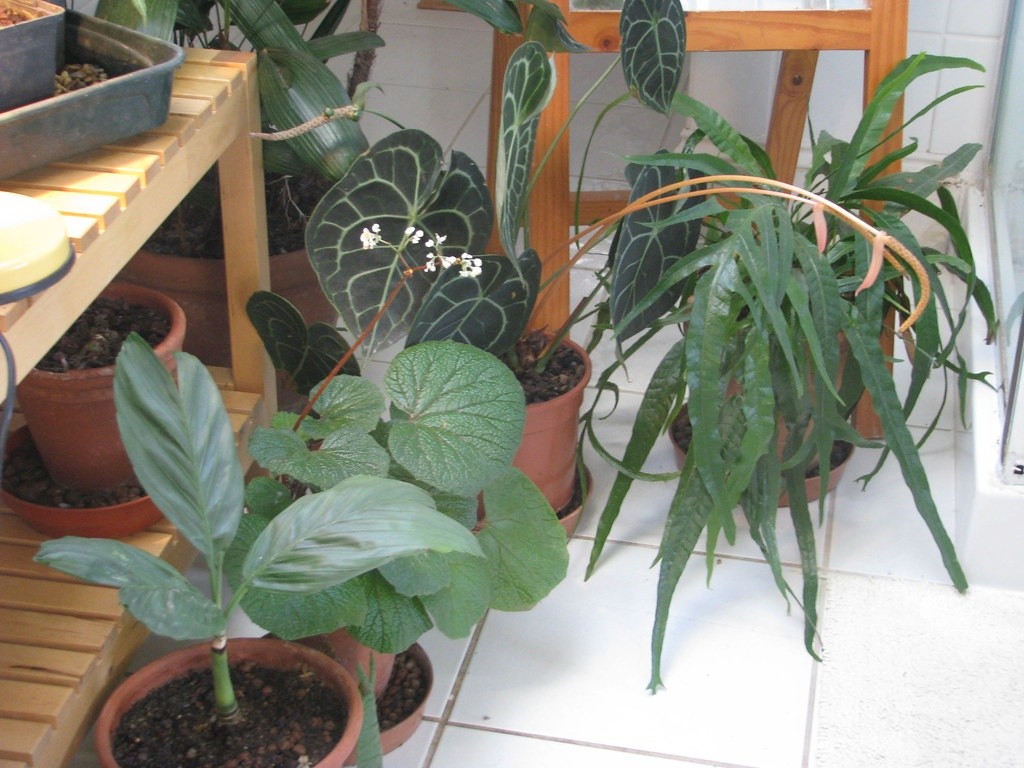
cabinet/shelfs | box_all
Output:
[0,48,280,768]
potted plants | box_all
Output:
[17,1,998,767]
